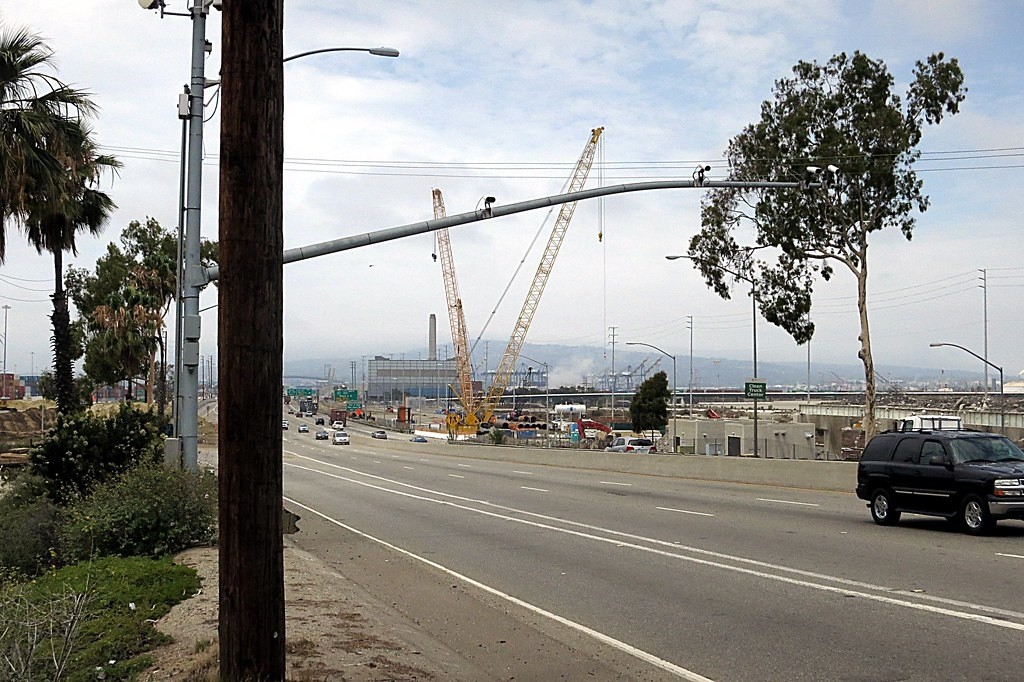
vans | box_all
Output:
[315,418,324,425]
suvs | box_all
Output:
[297,424,309,433]
[371,431,388,440]
[604,437,657,454]
[306,411,312,417]
[855,428,1023,533]
[332,432,351,444]
[179,47,402,468]
[332,420,344,431]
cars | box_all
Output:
[288,409,293,414]
[316,430,329,440]
[409,435,427,443]
[296,412,304,418]
[282,419,289,429]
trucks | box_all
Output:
[329,408,347,427]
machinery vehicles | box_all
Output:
[576,418,614,449]
[0,440,39,481]
[447,384,496,440]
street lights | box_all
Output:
[505,352,550,447]
[625,342,677,453]
[361,380,393,429]
[3,304,11,398]
[666,255,757,457]
[393,377,421,427]
[930,343,1004,436]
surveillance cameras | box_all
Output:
[807,166,822,174]
[485,197,496,203]
[828,165,840,174]
[699,161,711,171]
